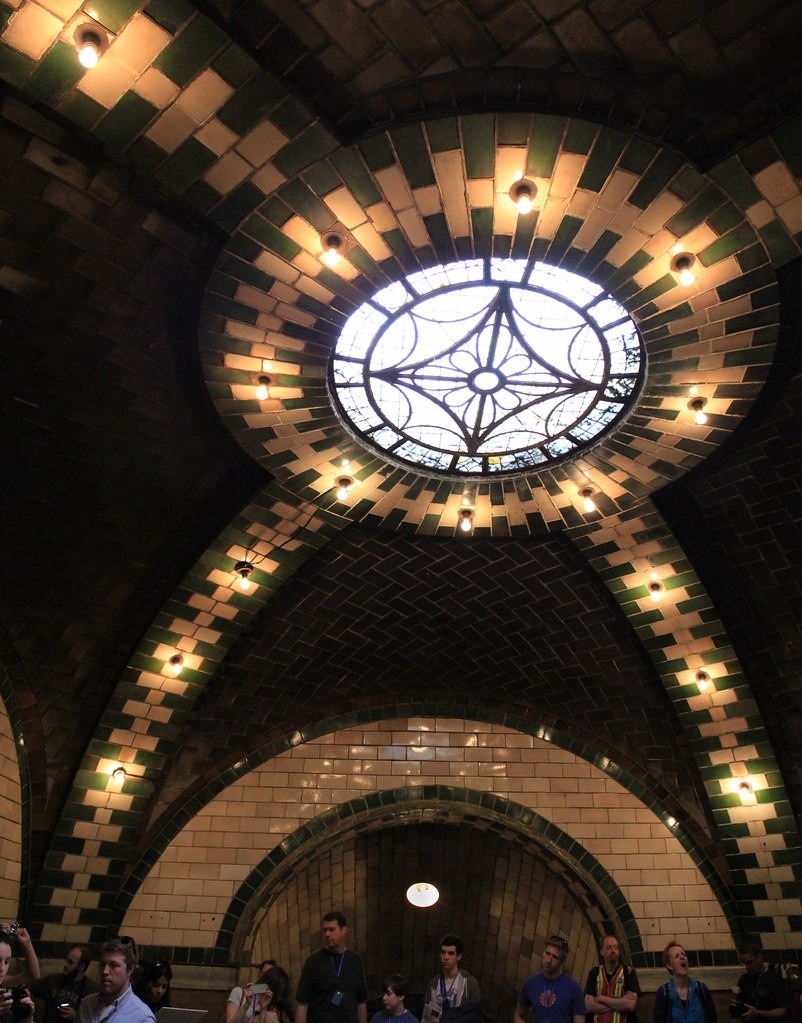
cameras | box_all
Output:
[4,984,31,1021]
[9,922,19,936]
[728,1001,757,1019]
[51,998,76,1020]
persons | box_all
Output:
[583,933,643,1022]
[224,967,290,1022]
[728,936,793,1023]
[514,935,587,1023]
[294,912,371,1022]
[369,973,420,1023]
[75,936,159,1023]
[0,930,36,1022]
[424,934,483,1023]
[130,950,173,1015]
[438,1006,495,1023]
[651,940,719,1023]
[28,942,102,1023]
[1,920,47,1023]
[257,959,292,1023]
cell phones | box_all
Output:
[250,983,268,993]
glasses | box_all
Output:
[111,935,137,957]
[550,935,568,946]
